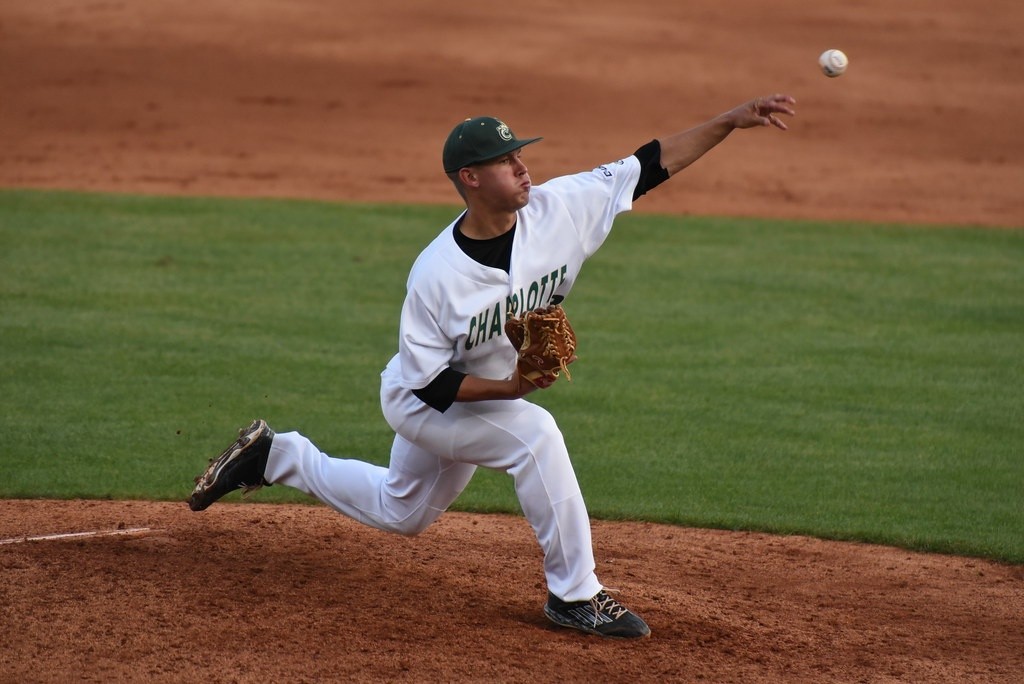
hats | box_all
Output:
[442,116,544,172]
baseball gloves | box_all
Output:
[504,307,577,391]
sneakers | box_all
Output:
[543,586,651,642]
[190,418,274,511]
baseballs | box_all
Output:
[818,49,848,78]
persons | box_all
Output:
[189,94,796,640]
